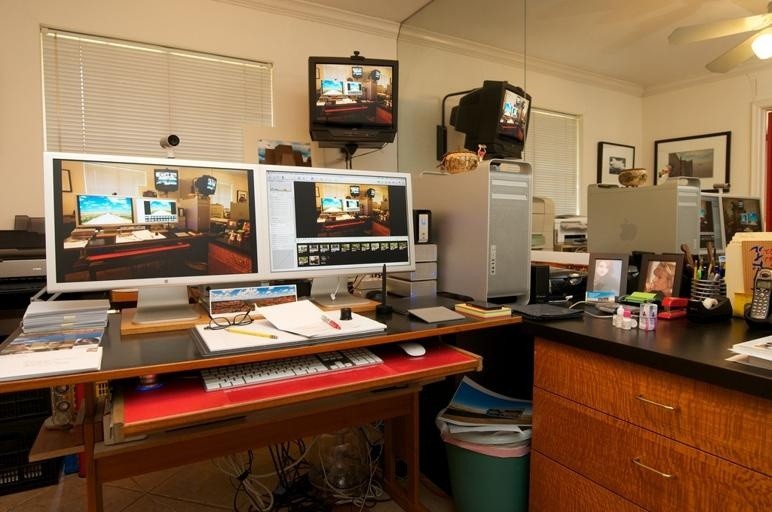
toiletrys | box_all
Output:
[621,305,632,330]
[612,307,618,326]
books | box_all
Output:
[722,230,772,300]
[188,299,388,357]
[725,335,772,371]
[0,299,111,382]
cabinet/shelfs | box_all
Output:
[528,318,772,511]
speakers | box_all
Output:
[530,263,551,303]
[50,385,74,425]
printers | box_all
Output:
[553,215,588,245]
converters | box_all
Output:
[278,477,305,502]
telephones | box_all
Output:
[744,266,771,327]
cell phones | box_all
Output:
[465,300,502,310]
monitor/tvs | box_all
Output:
[154,168,179,192]
[306,55,401,145]
[40,148,265,326]
[197,174,217,197]
[700,192,725,255]
[73,193,136,237]
[367,188,375,198]
[721,194,765,248]
[504,102,511,115]
[321,81,344,95]
[352,67,362,77]
[258,160,418,308]
[517,96,520,104]
[371,70,380,79]
[343,199,361,216]
[513,107,519,116]
[453,79,534,165]
[350,185,360,196]
[134,196,179,232]
[345,80,362,96]
[320,197,343,217]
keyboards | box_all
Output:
[199,348,384,393]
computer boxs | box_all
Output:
[415,158,536,310]
[586,176,702,254]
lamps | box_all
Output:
[752,30,772,60]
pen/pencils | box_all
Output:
[693,253,724,302]
[322,316,341,330]
[227,329,278,339]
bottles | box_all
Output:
[623,307,631,330]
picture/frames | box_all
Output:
[653,131,731,193]
[596,141,636,188]
[637,253,685,310]
[586,251,629,302]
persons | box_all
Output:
[594,260,618,293]
[514,97,526,122]
[646,261,675,298]
[725,200,747,226]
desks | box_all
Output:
[0,288,524,511]
[554,241,587,252]
[531,249,590,272]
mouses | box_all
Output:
[398,341,427,358]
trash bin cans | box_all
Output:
[439,413,530,510]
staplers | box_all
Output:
[658,298,698,320]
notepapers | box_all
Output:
[625,291,658,304]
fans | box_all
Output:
[667,1,772,74]
